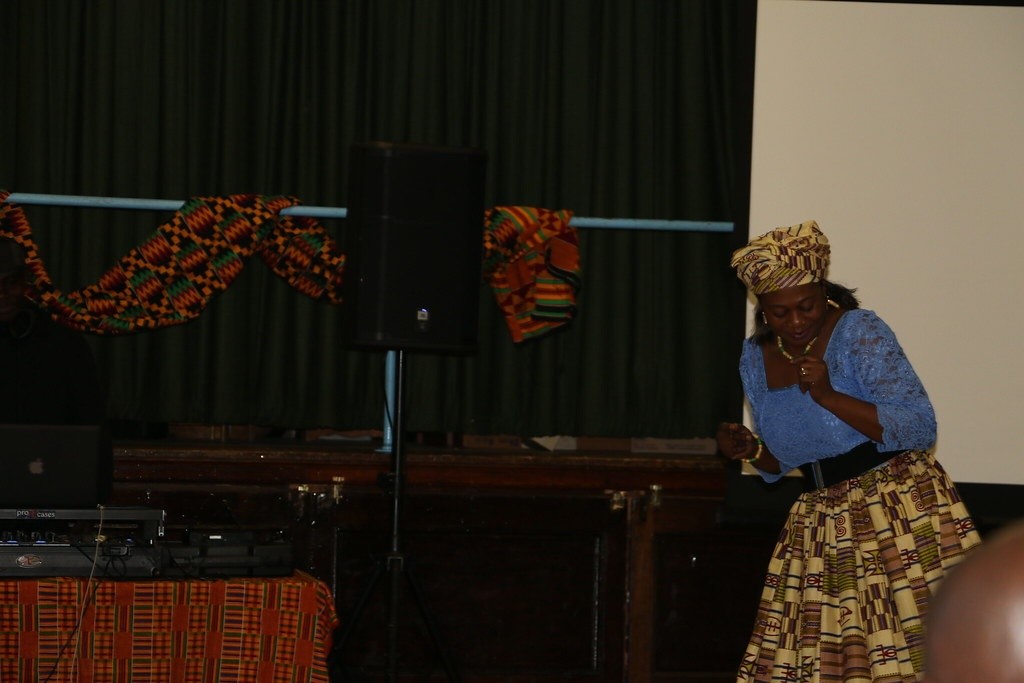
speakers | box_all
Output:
[343,139,487,358]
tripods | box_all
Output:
[328,350,461,681]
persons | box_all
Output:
[925,523,1024,683]
[716,221,986,683]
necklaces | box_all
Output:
[777,335,819,359]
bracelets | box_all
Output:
[740,432,762,463]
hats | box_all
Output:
[730,220,831,294]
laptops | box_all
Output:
[0,425,113,509]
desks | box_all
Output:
[0,571,343,682]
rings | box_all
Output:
[800,367,808,376]
